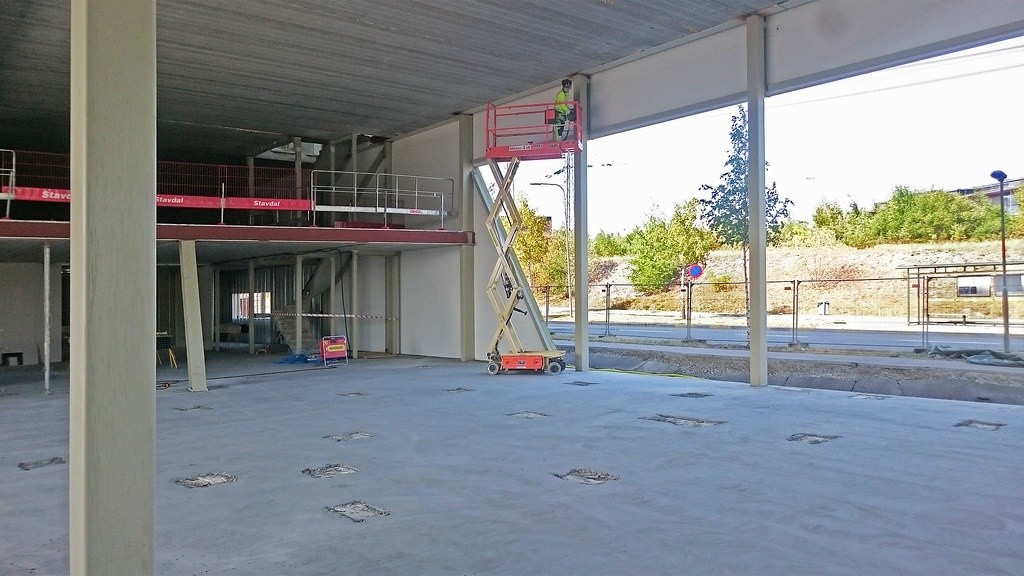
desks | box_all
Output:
[156,334,177,368]
[214,324,249,351]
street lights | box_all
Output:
[990,170,1010,353]
[530,182,572,317]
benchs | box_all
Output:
[926,312,969,325]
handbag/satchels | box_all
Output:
[567,109,576,121]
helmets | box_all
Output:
[562,79,572,87]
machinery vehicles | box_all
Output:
[485,101,584,375]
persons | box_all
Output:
[554,79,572,140]
